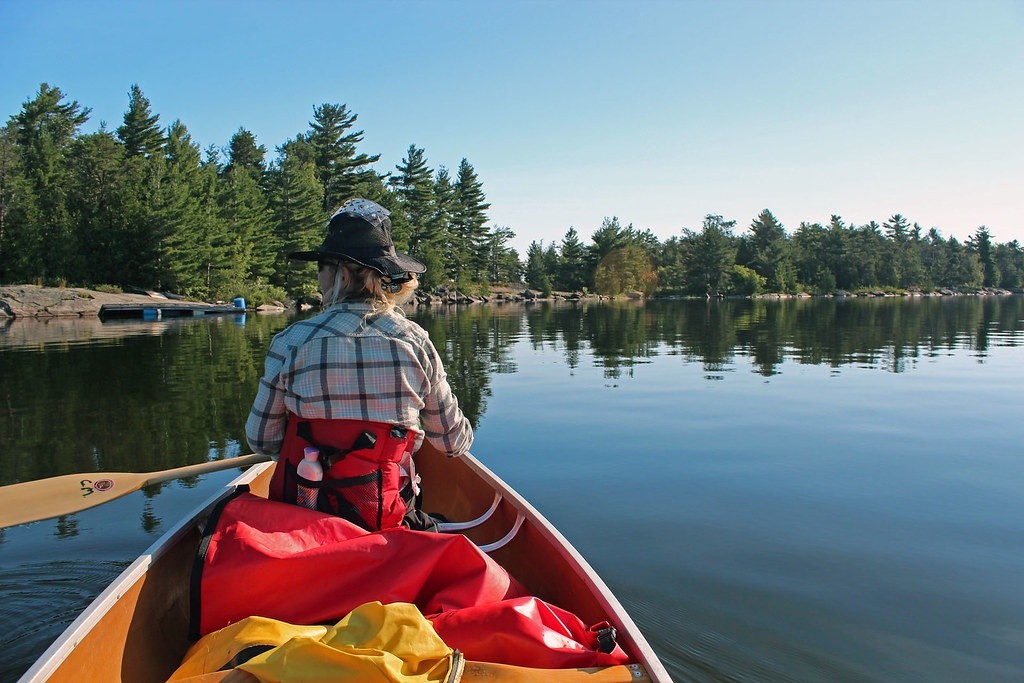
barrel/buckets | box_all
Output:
[234,298,245,309]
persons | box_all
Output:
[245,198,473,512]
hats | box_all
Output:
[284,198,427,276]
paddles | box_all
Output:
[0,455,273,529]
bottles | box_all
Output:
[297,446,323,510]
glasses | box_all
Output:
[317,259,338,273]
